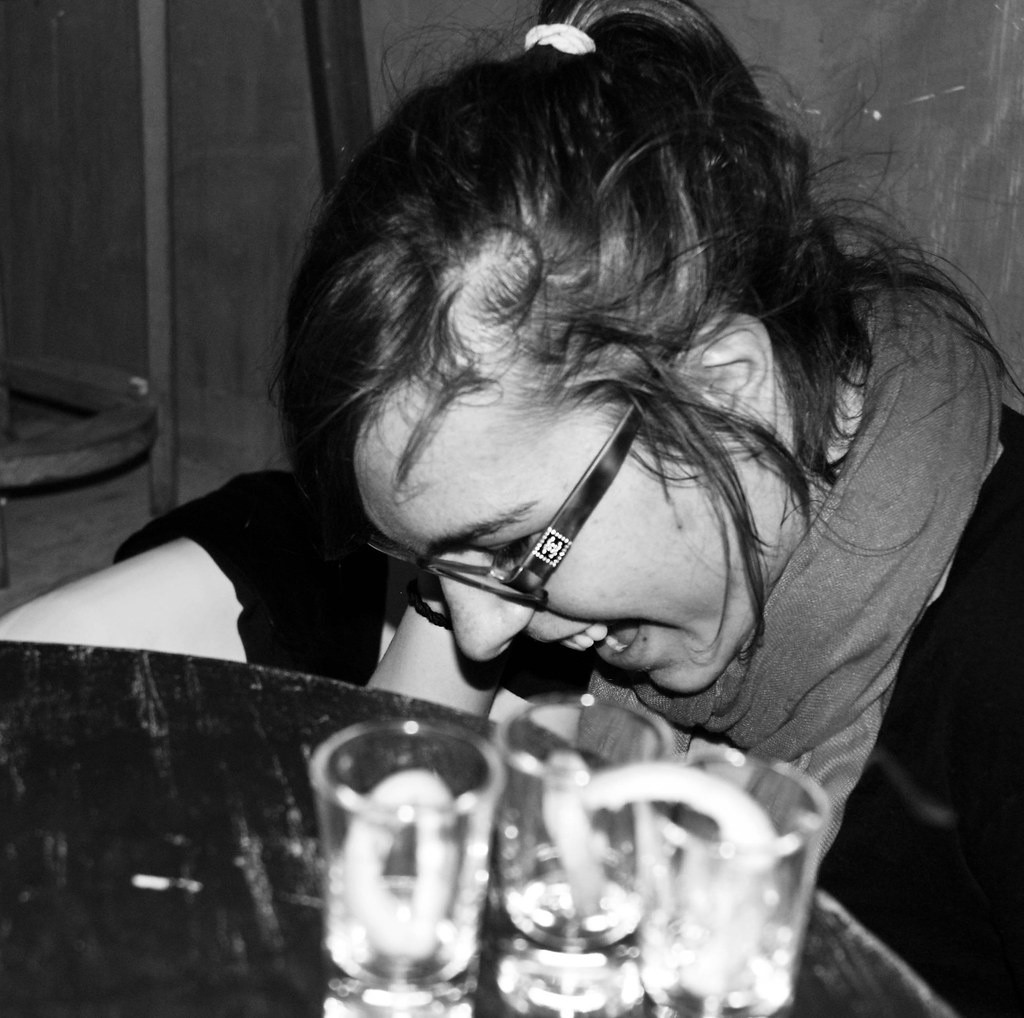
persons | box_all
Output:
[0,0,1024,1018]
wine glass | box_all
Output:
[310,697,831,1018]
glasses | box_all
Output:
[365,331,692,610]
